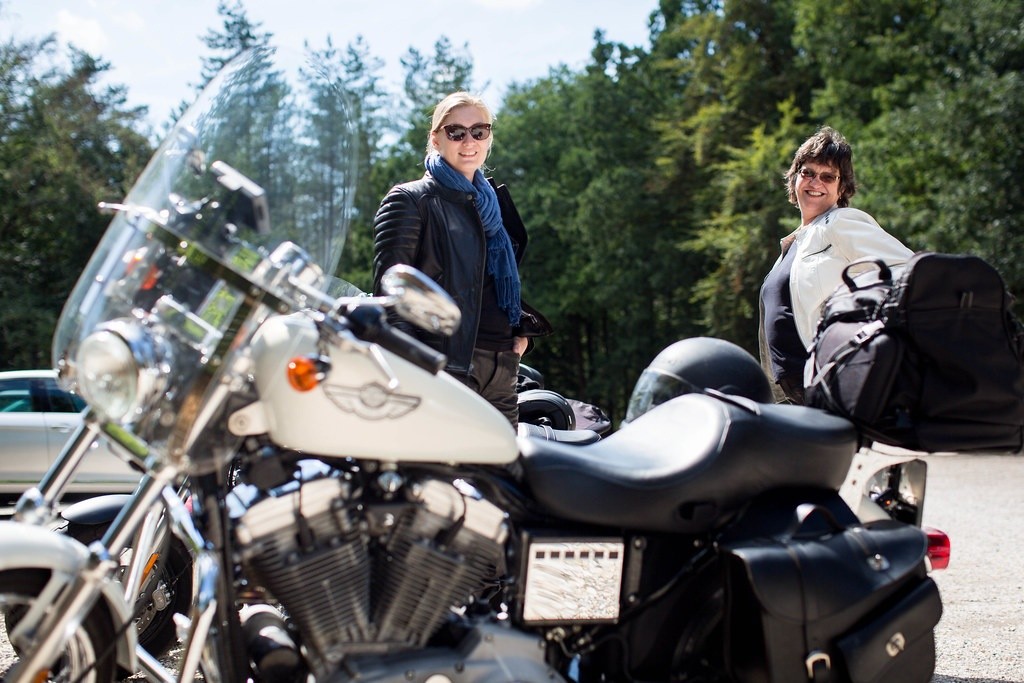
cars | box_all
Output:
[1,370,179,509]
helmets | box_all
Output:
[621,337,775,427]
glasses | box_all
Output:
[436,123,492,142]
[798,168,842,185]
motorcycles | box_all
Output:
[0,41,951,683]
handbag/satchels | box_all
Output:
[810,251,1024,456]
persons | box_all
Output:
[758,125,914,408]
[372,92,552,439]
